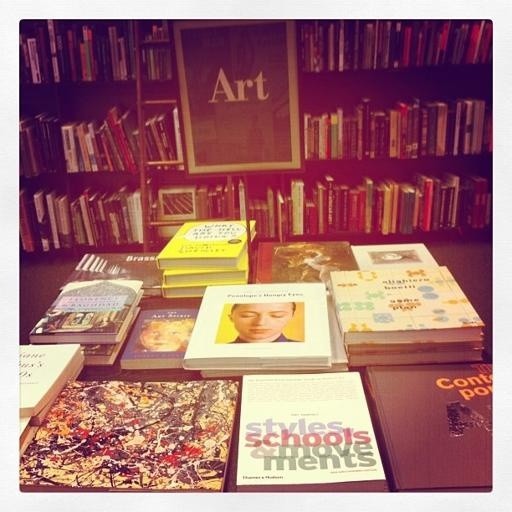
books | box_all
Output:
[302,20,492,236]
[363,361,492,493]
[19,20,186,251]
[231,370,388,492]
[19,342,81,420]
[30,220,487,378]
[199,177,305,244]
[20,379,240,492]
[20,415,39,457]
[28,357,87,427]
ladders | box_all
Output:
[134,20,235,254]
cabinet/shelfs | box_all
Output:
[20,19,493,267]
